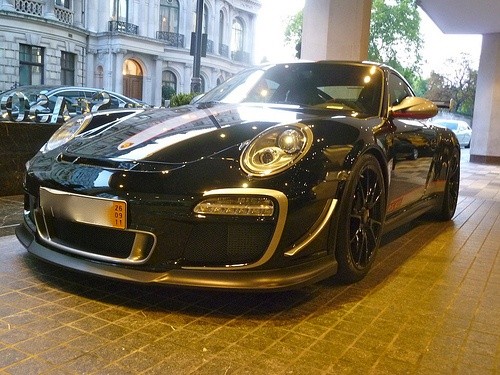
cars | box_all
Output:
[431,118,472,148]
[0,83,143,124]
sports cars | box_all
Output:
[14,59,462,298]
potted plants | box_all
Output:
[163,85,175,108]
